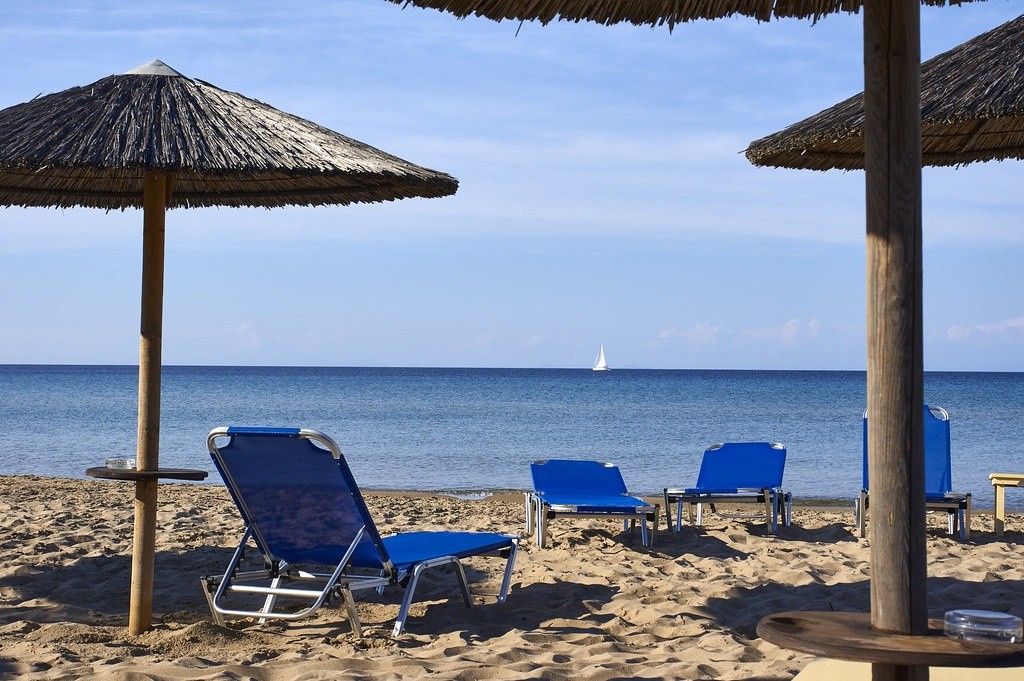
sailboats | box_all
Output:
[592,343,610,371]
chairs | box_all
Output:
[199,426,521,640]
[663,441,792,536]
[854,405,972,543]
[524,459,661,550]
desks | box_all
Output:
[86,467,209,481]
[756,611,1024,669]
[989,472,1024,538]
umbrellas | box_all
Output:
[0,59,459,636]
[388,1,1024,681]
